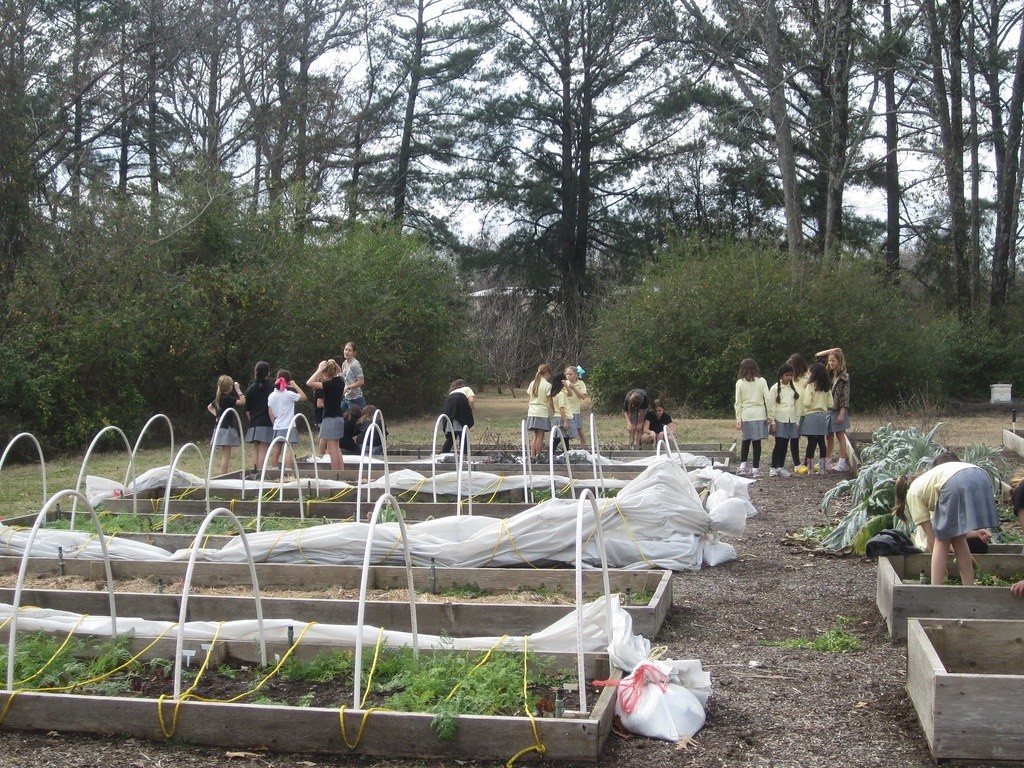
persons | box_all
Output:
[207,374,245,474]
[914,451,992,553]
[623,389,649,445]
[306,359,345,470]
[787,354,817,465]
[244,360,275,469]
[354,405,389,454]
[801,362,834,475]
[734,358,773,477]
[568,369,587,444]
[891,462,1000,586]
[339,404,362,455]
[1010,478,1024,598]
[815,348,851,472]
[341,341,366,413]
[551,373,579,453]
[441,379,476,460]
[312,367,326,455]
[770,364,800,478]
[268,369,308,470]
[641,398,675,444]
[526,364,555,456]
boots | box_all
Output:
[807,457,816,475]
[815,457,826,475]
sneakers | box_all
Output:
[747,468,759,477]
[778,467,792,477]
[832,462,846,471]
[795,464,807,474]
[736,466,746,475]
[816,461,830,470]
[769,466,779,477]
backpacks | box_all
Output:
[865,529,923,559]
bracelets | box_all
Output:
[348,385,350,390]
[238,393,244,397]
